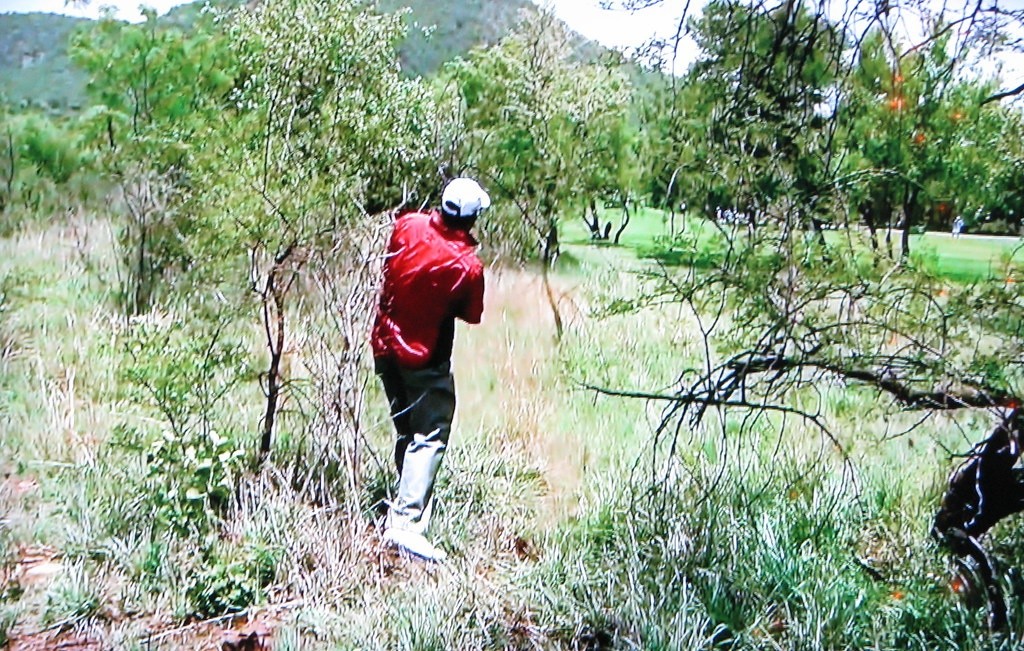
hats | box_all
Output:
[441,178,490,217]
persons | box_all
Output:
[371,178,490,559]
[952,216,965,238]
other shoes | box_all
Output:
[382,523,446,565]
